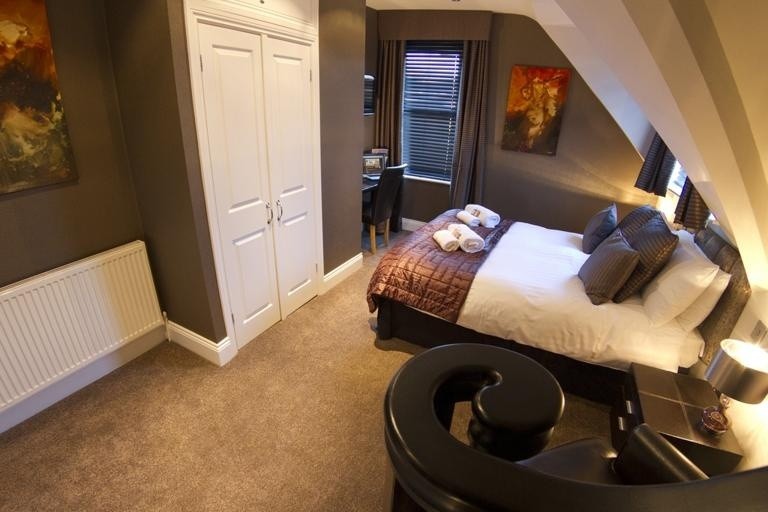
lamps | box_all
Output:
[700,339,768,437]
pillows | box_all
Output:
[611,204,678,304]
[676,269,733,333]
[577,228,640,305]
[640,229,720,330]
[582,202,618,253]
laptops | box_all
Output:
[362,153,386,181]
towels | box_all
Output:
[456,210,481,227]
[432,230,460,253]
[448,224,485,254]
[465,204,501,228]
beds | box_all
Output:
[367,207,751,407]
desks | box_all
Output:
[362,172,403,232]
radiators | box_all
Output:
[0,240,165,413]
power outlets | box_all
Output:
[751,320,768,345]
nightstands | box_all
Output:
[610,362,744,477]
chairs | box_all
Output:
[362,163,408,254]
[384,343,767,512]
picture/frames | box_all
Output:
[363,154,386,174]
